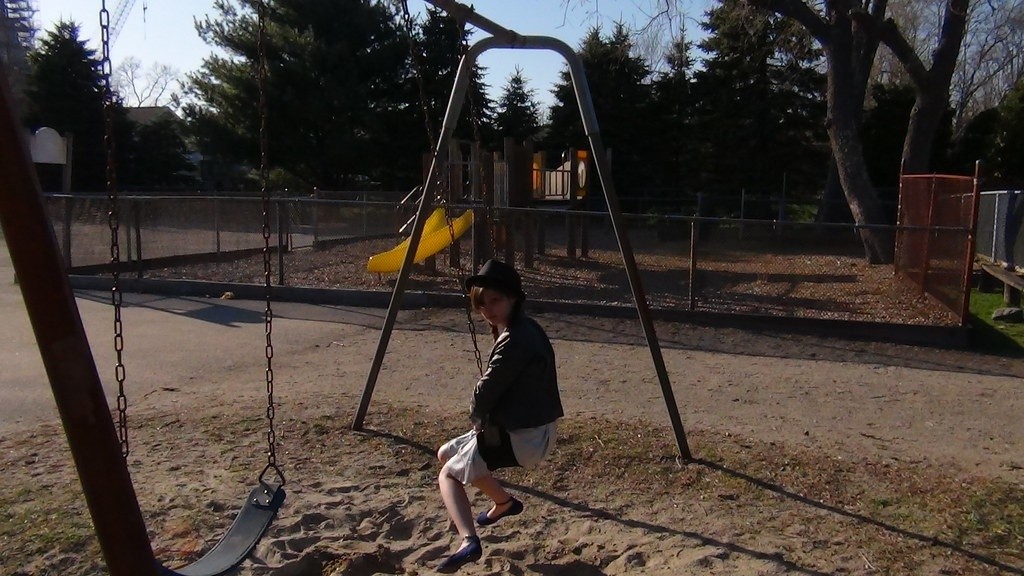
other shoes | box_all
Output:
[476,497,524,526]
[436,536,482,574]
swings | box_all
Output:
[399,0,525,471]
[94,1,287,576]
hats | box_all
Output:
[465,259,526,304]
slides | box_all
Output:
[366,206,473,272]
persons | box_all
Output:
[436,260,563,576]
